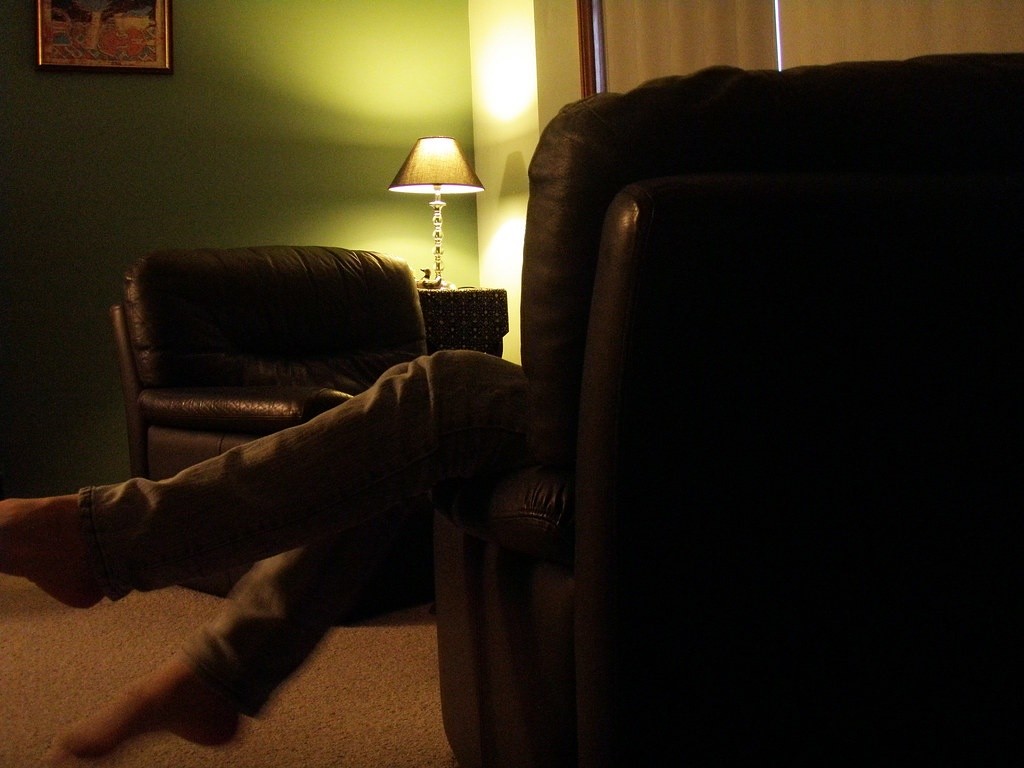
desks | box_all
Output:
[419,286,510,359]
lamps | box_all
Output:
[389,136,488,287]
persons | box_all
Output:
[1,352,530,758]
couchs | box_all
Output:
[435,51,1024,768]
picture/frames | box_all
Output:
[34,0,177,76]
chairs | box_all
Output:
[109,247,421,476]
